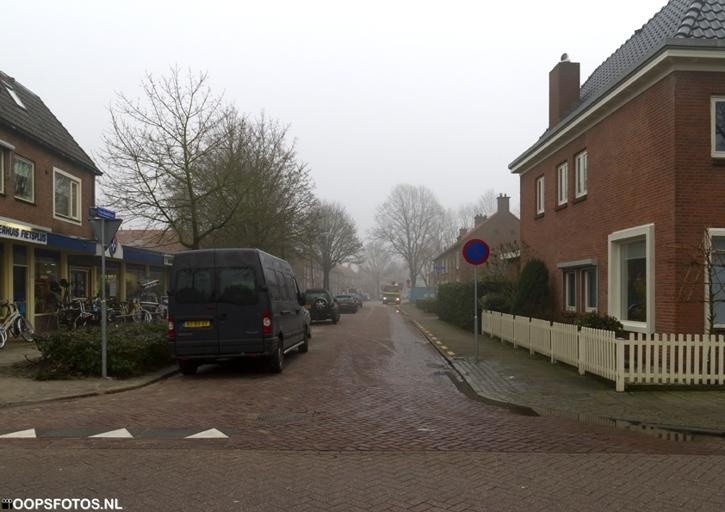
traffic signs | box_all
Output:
[97,205,116,222]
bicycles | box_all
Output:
[57,291,168,332]
[0,299,35,349]
[1,299,11,342]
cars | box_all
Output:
[336,289,370,314]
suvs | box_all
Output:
[304,287,341,324]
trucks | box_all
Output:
[168,247,311,375]
[382,285,401,305]
